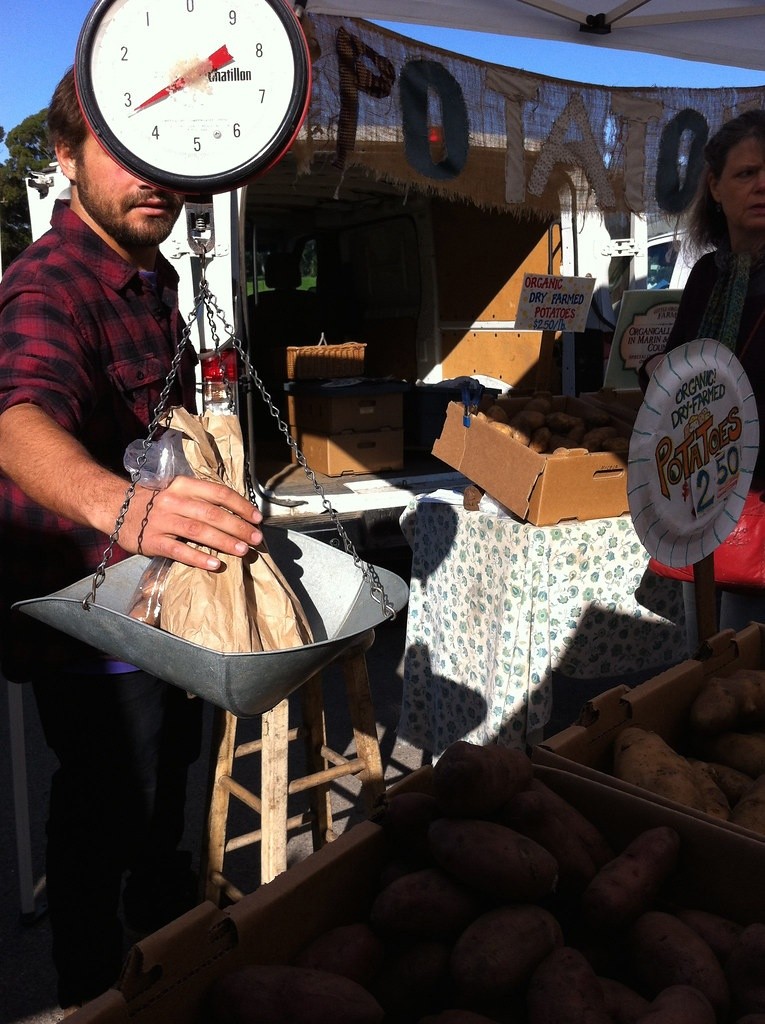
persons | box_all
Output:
[618,104,765,658]
[0,62,266,1018]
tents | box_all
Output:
[284,3,762,77]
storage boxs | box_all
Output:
[580,389,645,424]
[55,740,765,1024]
[294,397,403,433]
[431,393,639,527]
[532,621,765,840]
[298,429,404,478]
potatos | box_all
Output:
[212,739,765,1024]
[607,666,765,841]
[130,563,169,628]
[468,395,630,458]
[464,484,485,511]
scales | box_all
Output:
[11,0,412,718]
